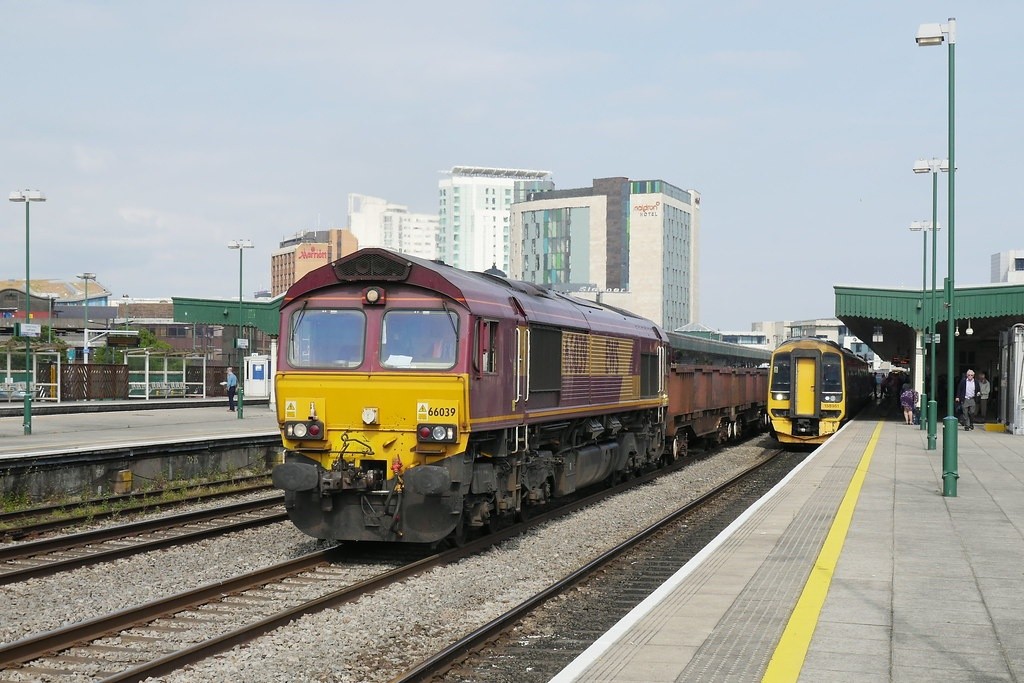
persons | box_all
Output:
[872,369,915,426]
[954,368,991,431]
[226,367,239,412]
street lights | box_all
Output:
[9,188,47,438]
[909,219,942,429]
[914,16,960,498]
[912,156,960,450]
[228,238,255,419]
[76,272,97,400]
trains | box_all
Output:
[766,335,885,445]
[272,247,773,552]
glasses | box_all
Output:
[967,375,974,376]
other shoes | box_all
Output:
[970,424,973,431]
[965,427,970,431]
[227,409,235,412]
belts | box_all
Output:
[967,397,974,399]
